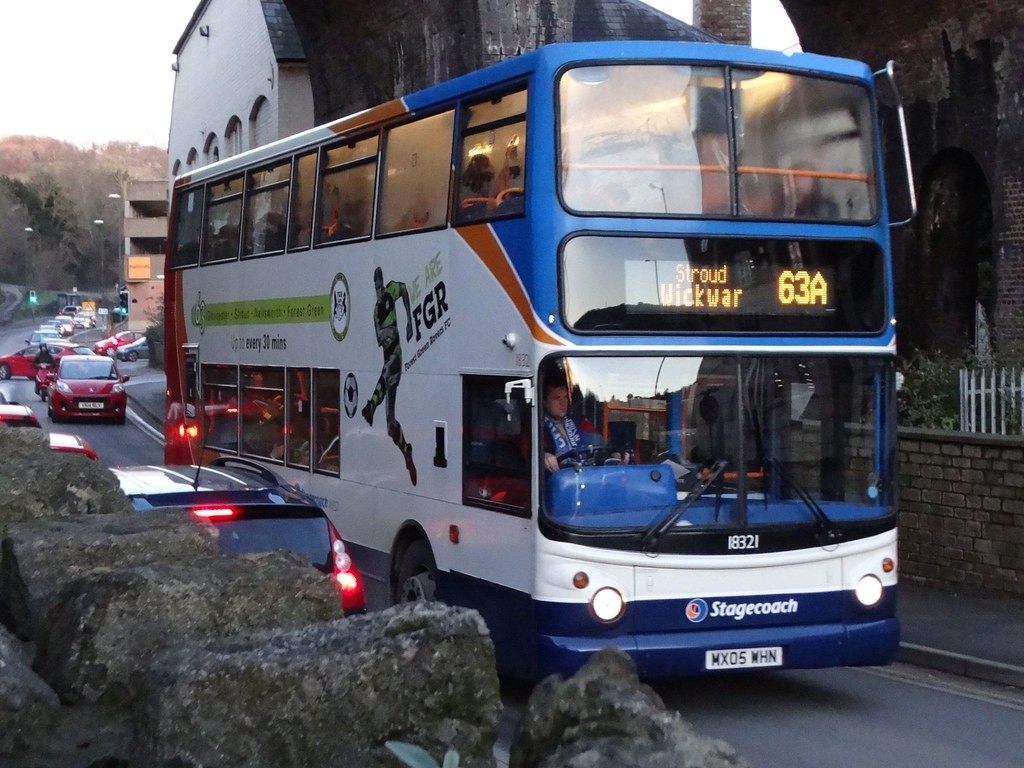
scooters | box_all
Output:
[31,361,55,402]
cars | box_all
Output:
[116,337,149,362]
[46,431,100,463]
[94,331,144,357]
[24,305,82,347]
[0,341,96,379]
[72,310,97,330]
[0,403,43,429]
[163,384,193,465]
[45,354,130,425]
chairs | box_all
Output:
[463,198,493,221]
[495,188,523,213]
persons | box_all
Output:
[767,164,829,219]
[361,266,417,486]
[300,441,340,473]
[228,373,283,456]
[318,192,351,244]
[524,381,631,473]
[457,158,491,216]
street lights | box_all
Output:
[93,219,106,289]
[24,227,32,286]
[110,192,124,288]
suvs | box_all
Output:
[112,454,384,614]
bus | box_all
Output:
[161,41,899,687]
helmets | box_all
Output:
[40,343,47,351]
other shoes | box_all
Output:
[35,387,40,394]
